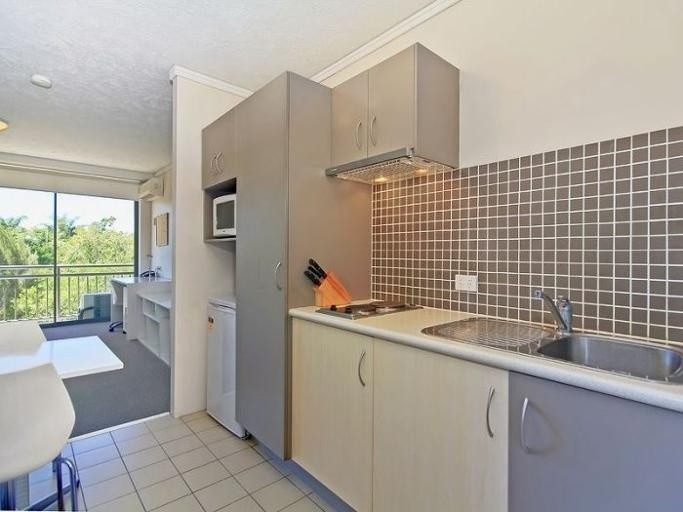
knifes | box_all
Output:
[304,257,327,286]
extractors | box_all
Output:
[336,157,454,186]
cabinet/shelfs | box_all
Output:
[235,70,372,465]
[136,291,172,367]
[291,319,372,512]
[371,336,683,512]
[201,106,237,243]
[326,41,460,178]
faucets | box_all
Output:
[533,290,573,335]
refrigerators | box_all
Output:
[207,296,243,439]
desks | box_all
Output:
[111,277,172,341]
[0,335,124,512]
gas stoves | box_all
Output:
[316,300,423,320]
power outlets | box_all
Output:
[455,275,478,292]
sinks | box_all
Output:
[538,330,683,386]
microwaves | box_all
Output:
[212,194,237,237]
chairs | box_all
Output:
[0,320,60,473]
[108,280,126,332]
[0,361,79,511]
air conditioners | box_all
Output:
[139,177,164,203]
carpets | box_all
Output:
[40,321,171,442]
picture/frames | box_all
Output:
[156,212,169,247]
